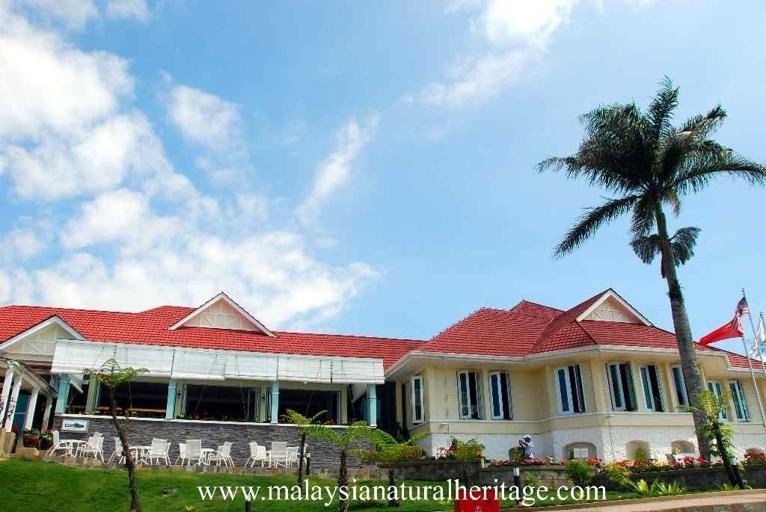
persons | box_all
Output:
[519,433,534,459]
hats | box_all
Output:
[523,436,531,442]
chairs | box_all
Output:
[44,429,308,471]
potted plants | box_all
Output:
[22,427,52,450]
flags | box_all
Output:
[748,311,765,358]
[728,295,753,334]
[698,319,743,346]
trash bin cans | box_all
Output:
[454,488,500,512]
